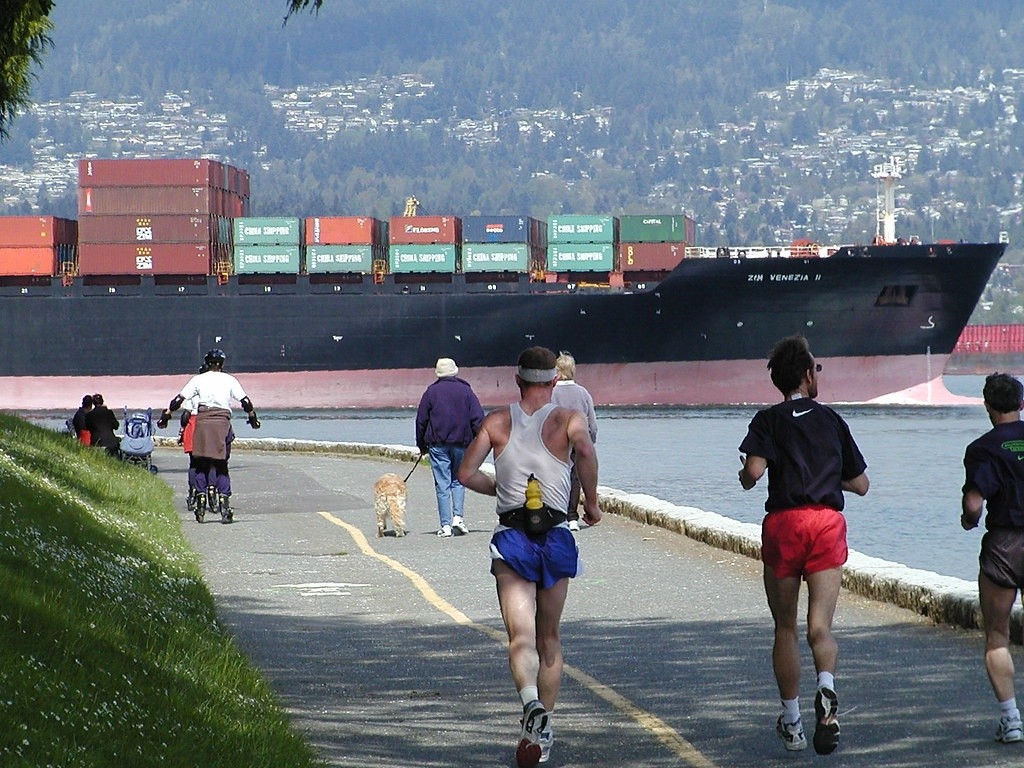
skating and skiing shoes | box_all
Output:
[208,485,218,512]
[187,487,198,511]
[218,493,233,524]
[193,490,206,522]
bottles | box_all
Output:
[525,473,544,509]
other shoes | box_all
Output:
[568,520,580,532]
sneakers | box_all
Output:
[436,524,451,537]
[452,517,468,534]
[994,715,1023,742]
[539,729,554,762]
[516,701,548,768]
[813,686,840,755]
[777,711,808,751]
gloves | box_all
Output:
[246,413,260,429]
[157,409,172,428]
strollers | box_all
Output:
[116,404,158,475]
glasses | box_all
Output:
[809,364,822,372]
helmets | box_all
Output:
[198,365,209,372]
[204,349,225,363]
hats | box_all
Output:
[435,357,458,377]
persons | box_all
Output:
[177,363,218,513]
[458,347,603,768]
[157,349,261,523]
[739,335,869,755]
[73,395,93,439]
[415,358,484,538]
[84,394,120,457]
[551,352,597,531]
[962,373,1024,743]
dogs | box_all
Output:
[370,471,411,539]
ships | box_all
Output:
[1,160,1009,405]
